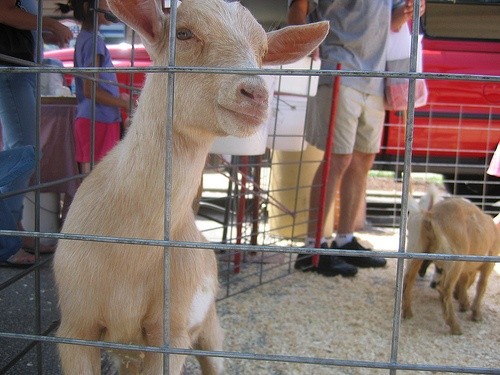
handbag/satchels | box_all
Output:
[384,21,428,111]
[29,97,80,197]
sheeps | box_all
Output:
[401,182,500,335]
[49,1,329,375]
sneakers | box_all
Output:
[331,237,386,268]
[295,242,357,276]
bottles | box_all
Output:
[40,58,63,97]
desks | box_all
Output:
[0,95,80,196]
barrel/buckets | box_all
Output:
[206,55,278,157]
[20,192,58,243]
[267,55,322,152]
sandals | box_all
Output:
[0,248,41,268]
[22,238,56,255]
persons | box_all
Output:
[57,0,130,180]
[0,0,75,269]
[287,0,426,277]
